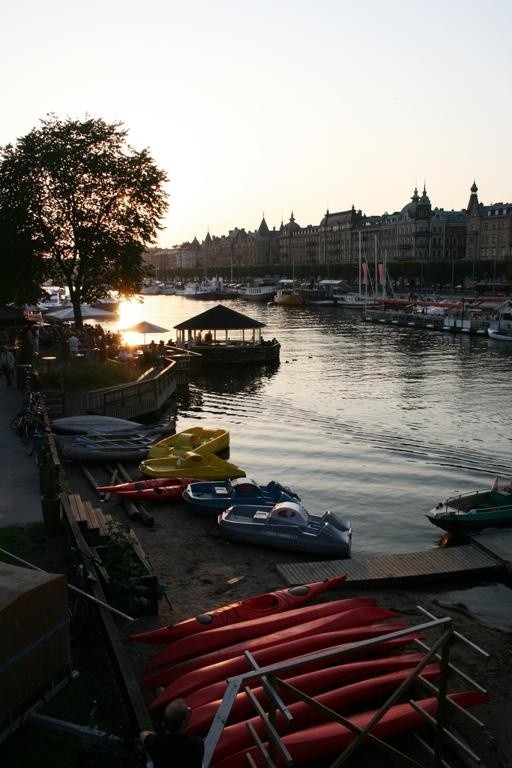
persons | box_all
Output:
[197,329,203,341]
[204,330,212,341]
[0,345,16,389]
[41,322,121,361]
[148,338,177,353]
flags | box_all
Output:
[377,263,385,285]
[362,262,370,285]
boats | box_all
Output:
[424,485,512,534]
[139,277,384,308]
[363,297,512,341]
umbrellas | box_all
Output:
[44,304,119,321]
[119,321,170,343]
